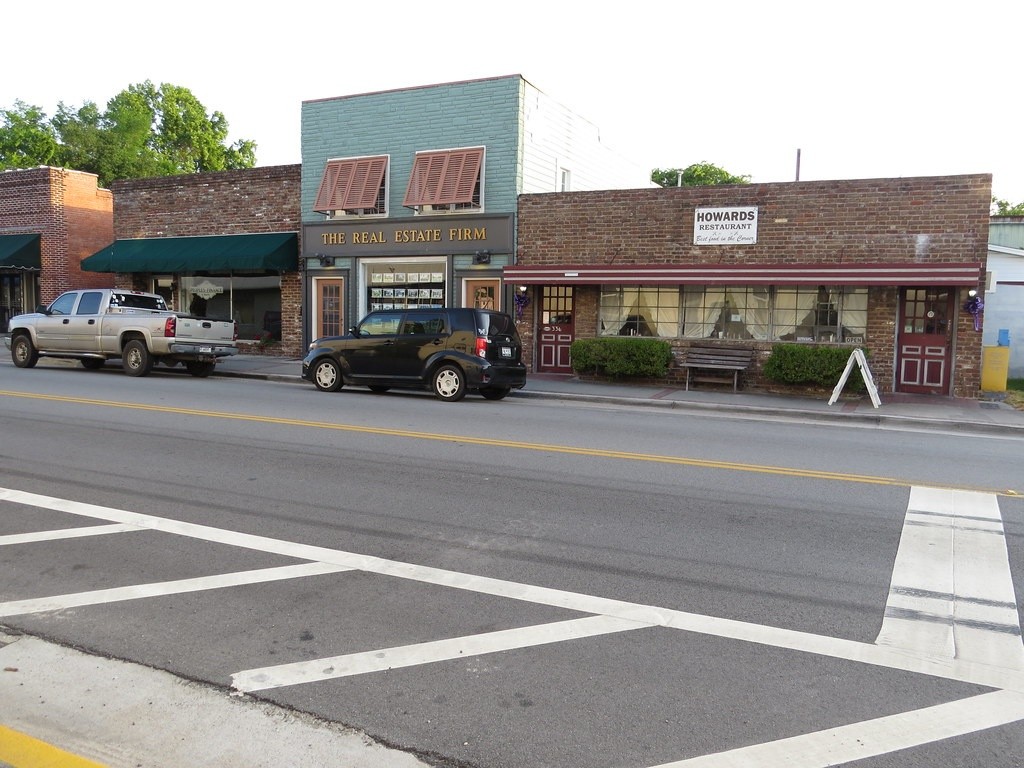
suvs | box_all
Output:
[301,308,528,402]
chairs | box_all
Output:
[407,323,425,334]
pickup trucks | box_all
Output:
[4,288,240,377]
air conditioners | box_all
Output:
[985,269,998,294]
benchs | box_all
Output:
[679,343,754,393]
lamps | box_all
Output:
[475,249,491,263]
[315,253,335,267]
